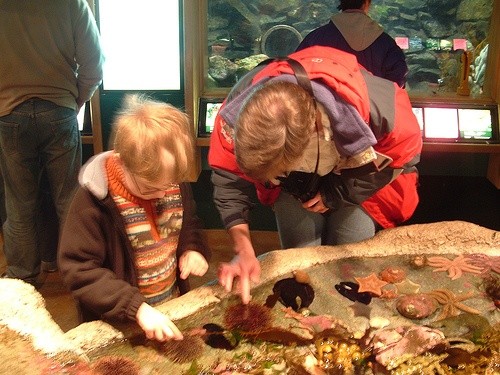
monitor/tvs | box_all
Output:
[196,96,499,144]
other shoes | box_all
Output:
[41,264,58,273]
[0,271,40,291]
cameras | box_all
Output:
[285,171,333,216]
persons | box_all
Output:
[57,95,212,343]
[293,1,410,90]
[0,0,106,286]
[208,45,423,305]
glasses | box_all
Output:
[130,172,172,196]
